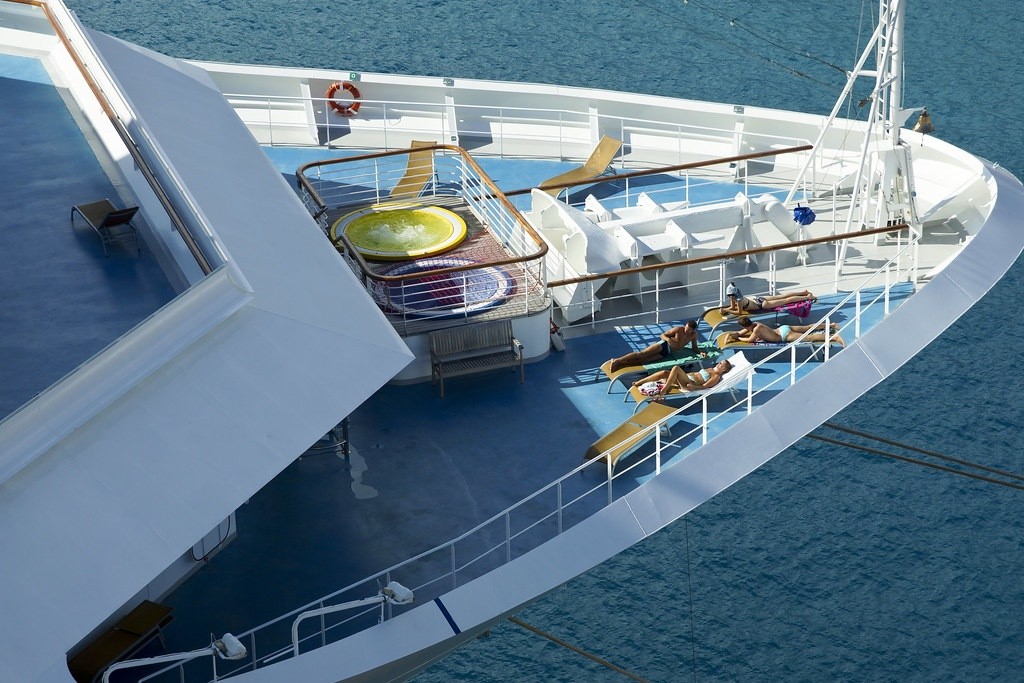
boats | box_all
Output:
[0,1,1023,683]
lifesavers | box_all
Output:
[325,81,362,116]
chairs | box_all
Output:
[713,331,832,361]
[624,351,756,416]
[595,341,723,394]
[538,134,624,198]
[71,198,141,256]
[388,140,440,199]
[696,299,816,341]
[579,401,678,480]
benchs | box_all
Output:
[68,600,175,683]
[428,318,524,400]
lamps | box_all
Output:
[293,581,414,656]
[102,635,248,683]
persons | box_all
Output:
[725,316,845,348]
[609,321,707,372]
[632,359,732,392]
[719,281,817,315]
